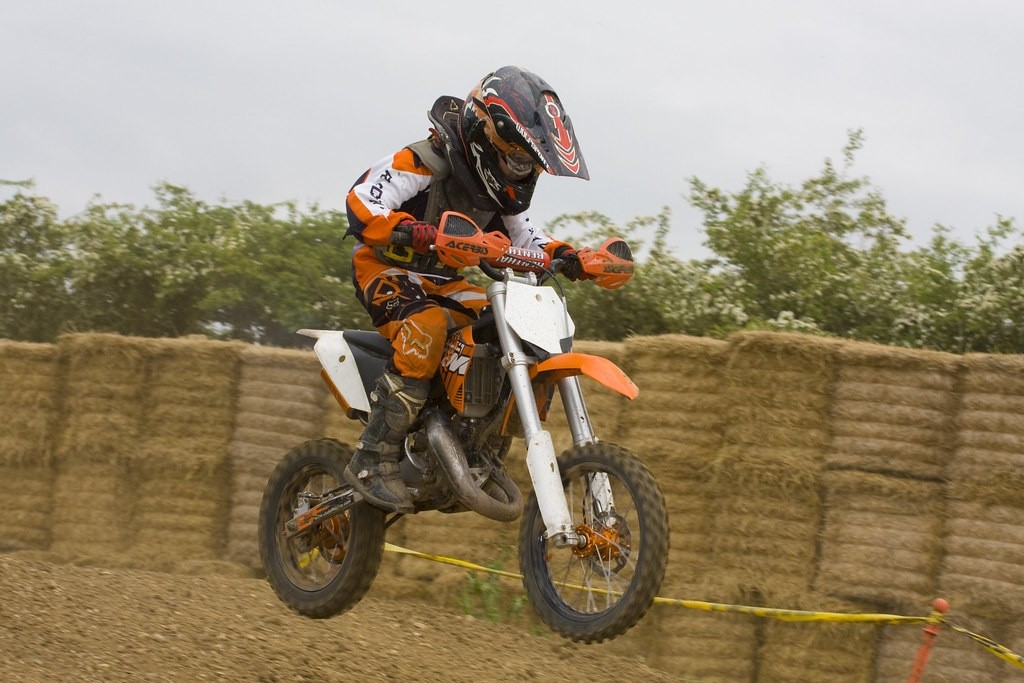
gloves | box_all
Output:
[553,247,596,282]
[396,220,439,256]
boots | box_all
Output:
[342,369,431,513]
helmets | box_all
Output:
[456,65,590,215]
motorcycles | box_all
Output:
[257,209,672,645]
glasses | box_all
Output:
[504,147,545,176]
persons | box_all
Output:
[343,66,597,513]
